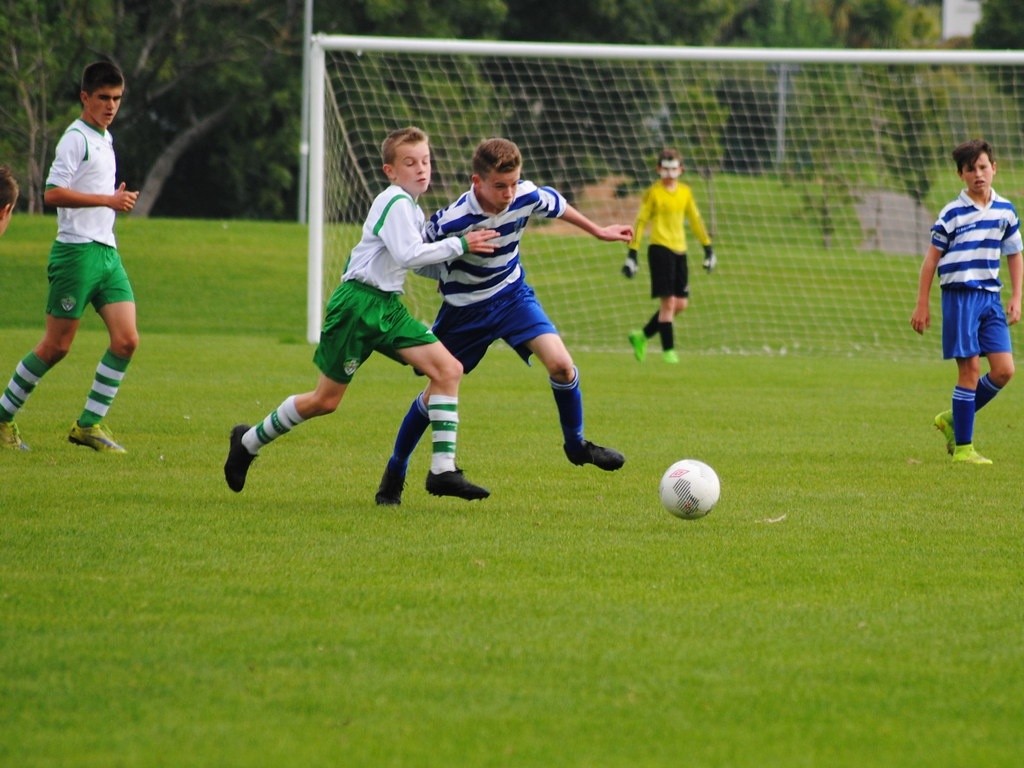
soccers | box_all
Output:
[659,459,721,519]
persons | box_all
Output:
[222,125,492,501]
[0,165,21,237]
[909,138,1024,466]
[622,148,717,365]
[376,136,637,505]
[0,57,146,453]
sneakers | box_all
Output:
[0,421,29,451]
[375,459,405,504]
[933,409,994,464]
[67,419,127,453]
[425,468,490,501]
[222,424,258,492]
[627,330,647,363]
[661,350,678,365]
[563,439,625,471]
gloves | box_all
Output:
[622,249,637,278]
[702,247,716,274]
